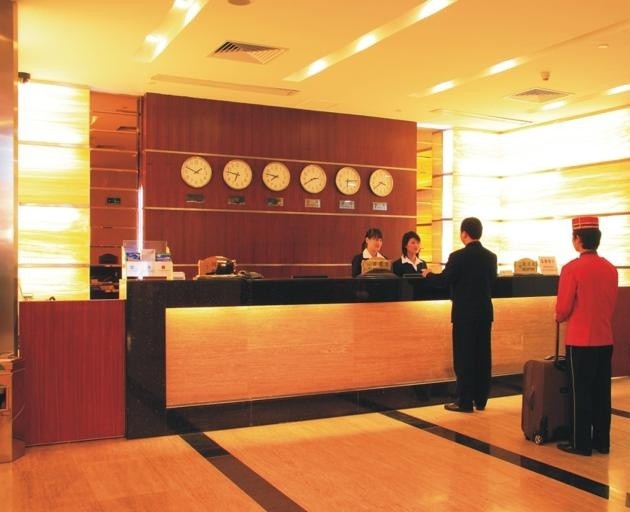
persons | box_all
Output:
[552,214,619,456]
[350,227,388,279]
[420,218,500,414]
[391,231,427,278]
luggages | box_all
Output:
[521,318,570,444]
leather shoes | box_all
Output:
[589,439,609,454]
[473,400,486,410]
[556,443,592,456]
[445,400,473,411]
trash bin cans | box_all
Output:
[0,353,26,463]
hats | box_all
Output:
[572,216,599,230]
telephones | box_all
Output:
[238,270,263,279]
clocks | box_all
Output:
[180,156,394,198]
